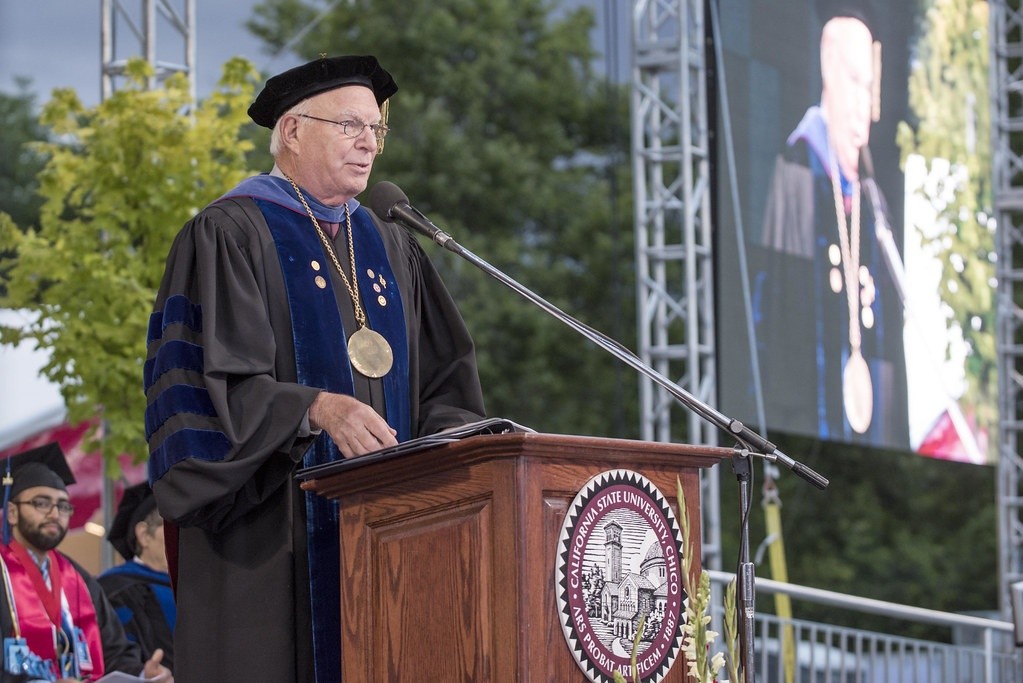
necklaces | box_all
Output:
[826,130,872,431]
[283,173,391,378]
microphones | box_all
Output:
[859,147,888,237]
[369,181,462,256]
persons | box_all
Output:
[143,54,485,683]
[753,16,909,450]
[0,440,174,683]
[95,480,174,677]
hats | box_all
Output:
[246,55,398,129]
[107,480,157,560]
[0,441,78,544]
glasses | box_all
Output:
[296,113,390,140]
[14,498,75,517]
